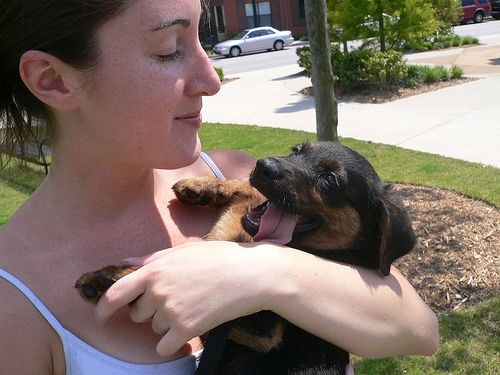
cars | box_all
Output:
[212,26,294,57]
[336,10,401,40]
[453,0,492,24]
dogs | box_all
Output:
[75,140,417,375]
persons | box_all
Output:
[0,0,439,375]
